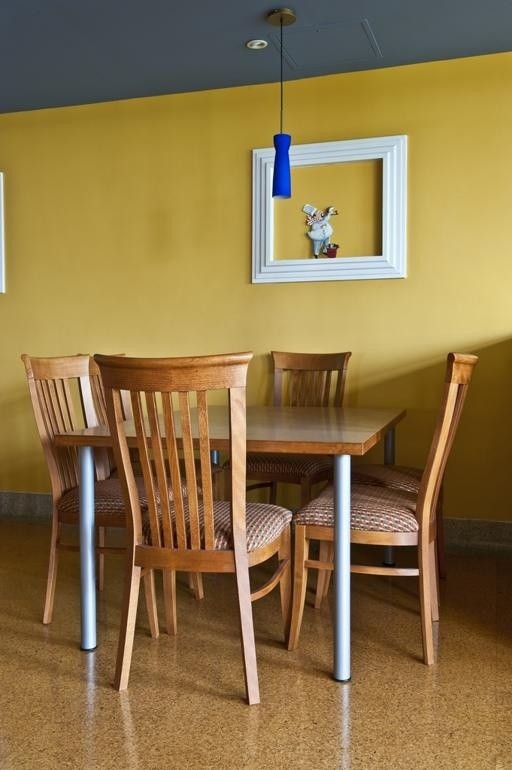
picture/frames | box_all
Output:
[0,172,5,294]
[250,133,409,286]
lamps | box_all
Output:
[264,8,297,197]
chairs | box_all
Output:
[285,351,479,666]
[323,463,447,607]
[78,352,222,591]
[22,353,204,641]
[92,352,292,705]
[220,350,358,507]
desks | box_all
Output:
[54,403,409,683]
